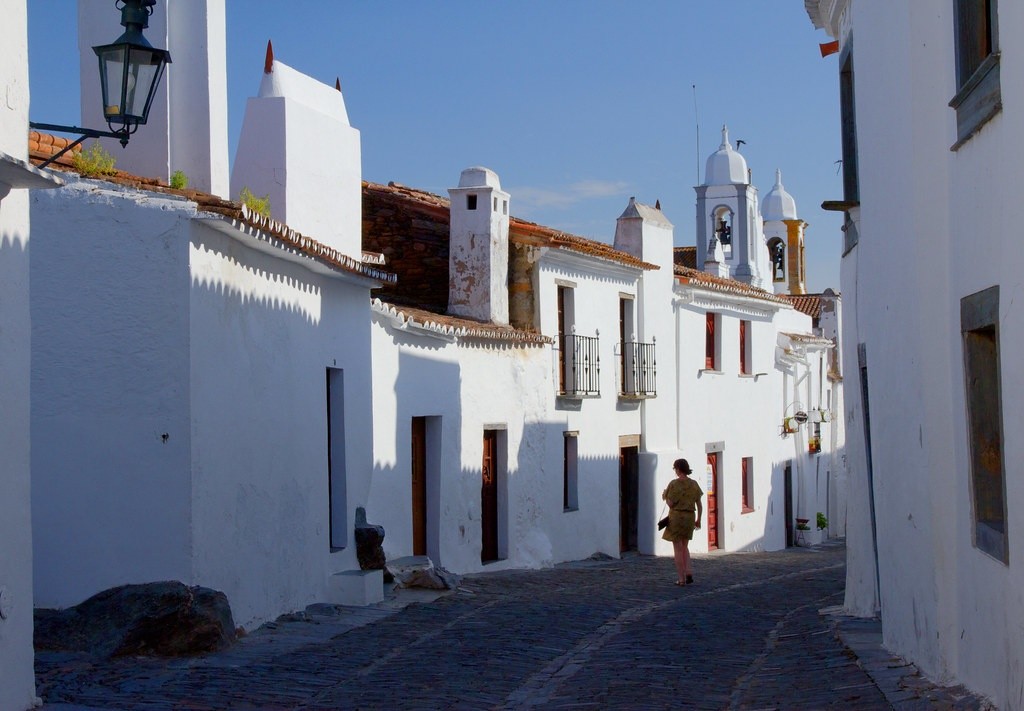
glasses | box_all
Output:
[673,467,676,470]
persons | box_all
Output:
[662,459,703,586]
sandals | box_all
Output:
[674,580,685,586]
[686,574,693,584]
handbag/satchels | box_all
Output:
[658,516,669,531]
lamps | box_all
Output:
[27,0,173,169]
